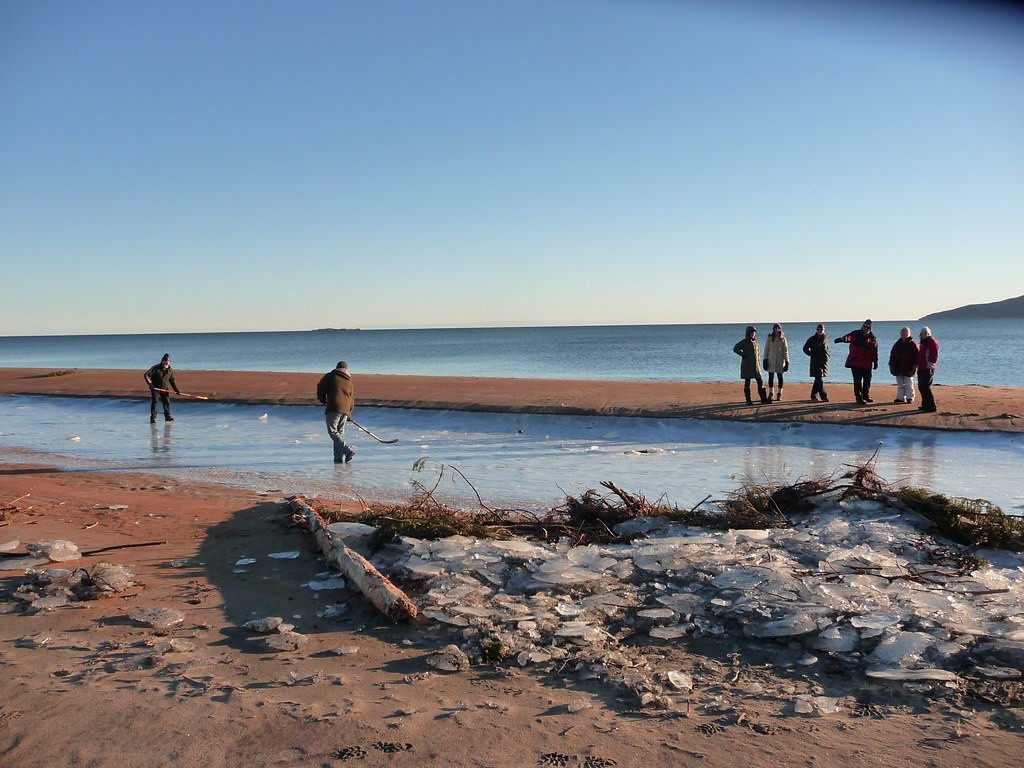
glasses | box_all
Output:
[863,325,871,329]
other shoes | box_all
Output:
[907,399,913,404]
[810,397,819,401]
[166,416,175,421]
[151,417,156,423]
[746,400,753,406]
[918,406,936,412]
[334,459,343,463]
[894,399,904,402]
[761,398,772,404]
[345,451,355,463]
[821,399,830,402]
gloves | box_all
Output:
[784,362,789,372]
[873,360,879,369]
[763,359,768,370]
[834,337,844,343]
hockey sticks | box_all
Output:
[154,387,216,400]
[350,420,399,444]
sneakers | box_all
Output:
[856,400,867,405]
[864,398,874,402]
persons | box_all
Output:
[918,327,939,412]
[834,319,878,405]
[888,327,919,404]
[803,324,830,402]
[733,326,772,405]
[317,361,356,463]
[144,353,180,423]
[763,324,789,401]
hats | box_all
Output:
[162,353,170,361]
[773,323,782,329]
[817,323,825,329]
[863,319,873,327]
[336,362,347,369]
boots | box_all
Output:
[777,393,782,401]
[768,393,775,400]
